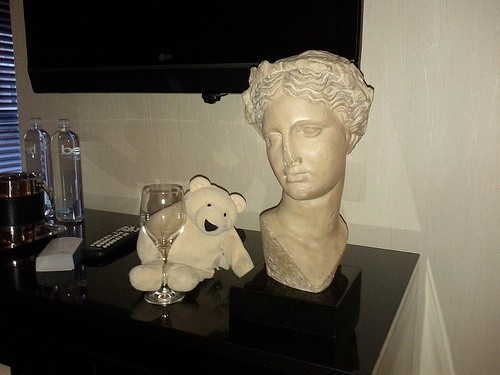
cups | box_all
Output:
[0,172,56,243]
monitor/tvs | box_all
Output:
[24,0,362,95]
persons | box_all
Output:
[241,49,375,294]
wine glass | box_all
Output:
[138,184,187,306]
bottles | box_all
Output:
[49,118,85,223]
[23,117,56,220]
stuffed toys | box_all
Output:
[128,174,254,292]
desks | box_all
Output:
[0,202,421,374]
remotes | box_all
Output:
[84,225,140,258]
